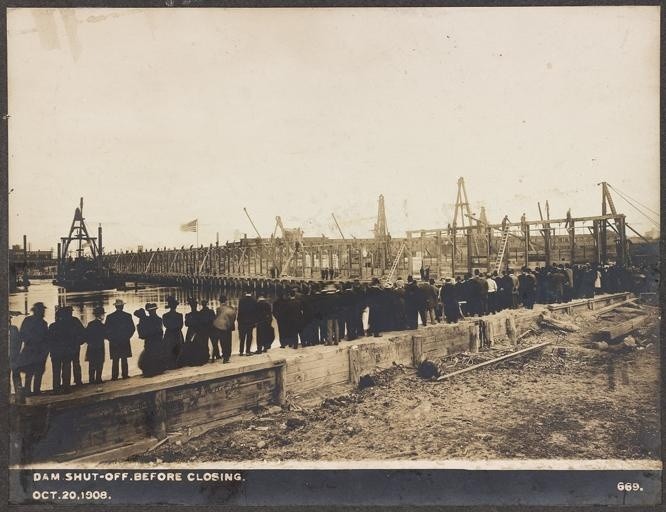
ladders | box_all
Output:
[494,222,510,274]
[386,244,405,282]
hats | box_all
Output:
[29,297,200,319]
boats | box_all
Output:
[51,196,117,291]
